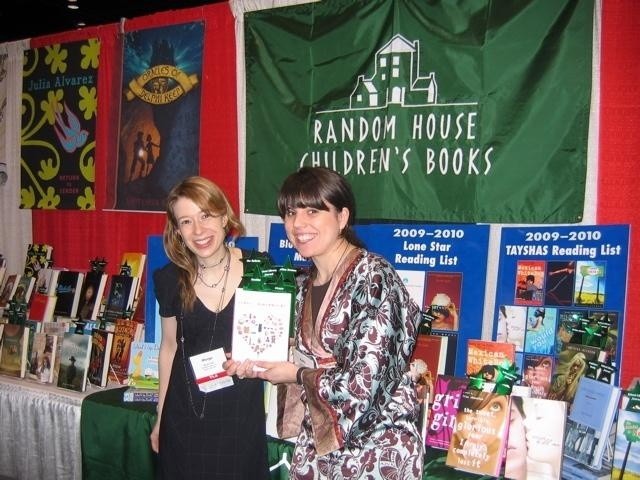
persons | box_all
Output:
[147,175,270,480]
[221,166,429,480]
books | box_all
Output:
[231,288,292,372]
[0,244,162,402]
[395,259,640,480]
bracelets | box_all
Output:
[296,367,308,384]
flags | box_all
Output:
[241,1,594,224]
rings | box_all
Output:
[239,375,245,379]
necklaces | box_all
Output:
[181,247,231,418]
[309,244,349,355]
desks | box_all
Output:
[1,374,510,479]
